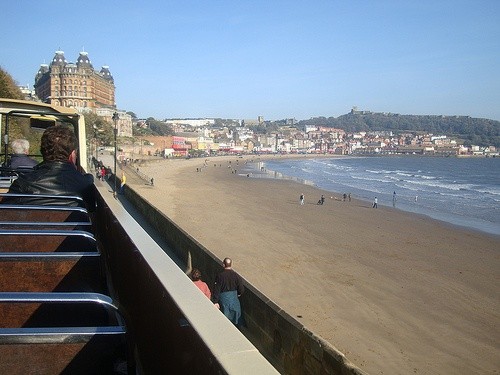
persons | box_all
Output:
[190,268,211,300]
[348,192,352,201]
[214,258,243,326]
[2,139,39,168]
[373,197,378,208]
[1,127,94,207]
[119,172,126,194]
[95,164,105,181]
[344,192,347,201]
[300,192,304,205]
[321,194,325,205]
[106,166,113,182]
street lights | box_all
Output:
[111,111,119,200]
[92,121,100,178]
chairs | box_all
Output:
[0,177,128,375]
